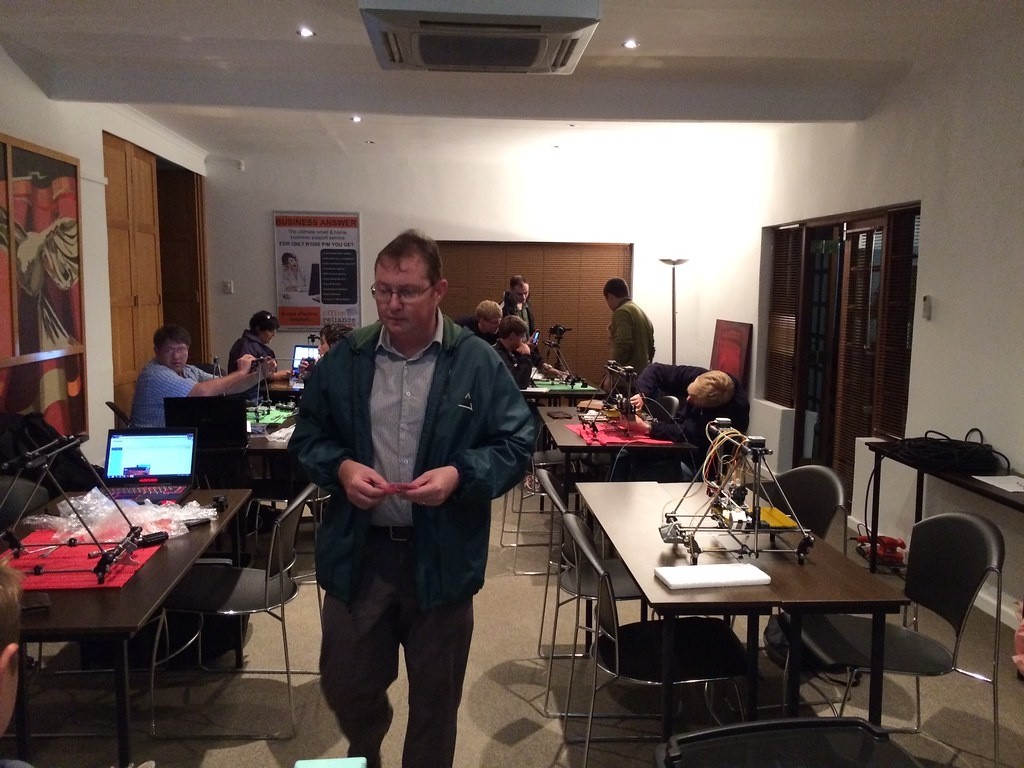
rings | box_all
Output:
[423,497,426,505]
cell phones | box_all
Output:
[533,331,540,344]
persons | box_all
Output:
[452,299,501,346]
[602,278,655,404]
[631,362,749,483]
[227,309,301,407]
[300,322,354,382]
[286,227,534,768]
[130,325,277,427]
[0,562,34,768]
[501,272,542,372]
[490,315,539,389]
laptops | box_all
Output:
[292,345,322,374]
[99,427,198,505]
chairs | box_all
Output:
[0,363,331,742]
[499,394,1005,768]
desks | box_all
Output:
[864,442,1024,680]
[519,375,608,408]
[194,415,322,556]
[258,379,305,404]
[0,489,253,768]
[537,407,698,576]
[575,482,912,744]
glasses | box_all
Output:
[266,314,275,320]
[370,280,435,299]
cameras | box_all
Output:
[550,324,565,336]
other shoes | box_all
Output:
[347,702,393,767]
